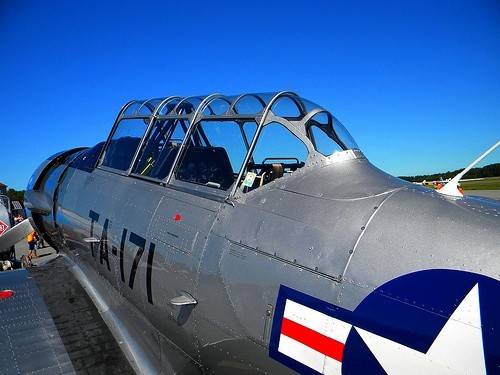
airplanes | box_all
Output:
[0,90,500,375]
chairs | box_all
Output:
[110,136,141,170]
[148,142,176,178]
[172,145,234,188]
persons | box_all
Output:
[0,260,11,271]
[13,214,24,226]
[436,181,463,195]
[26,231,45,262]
